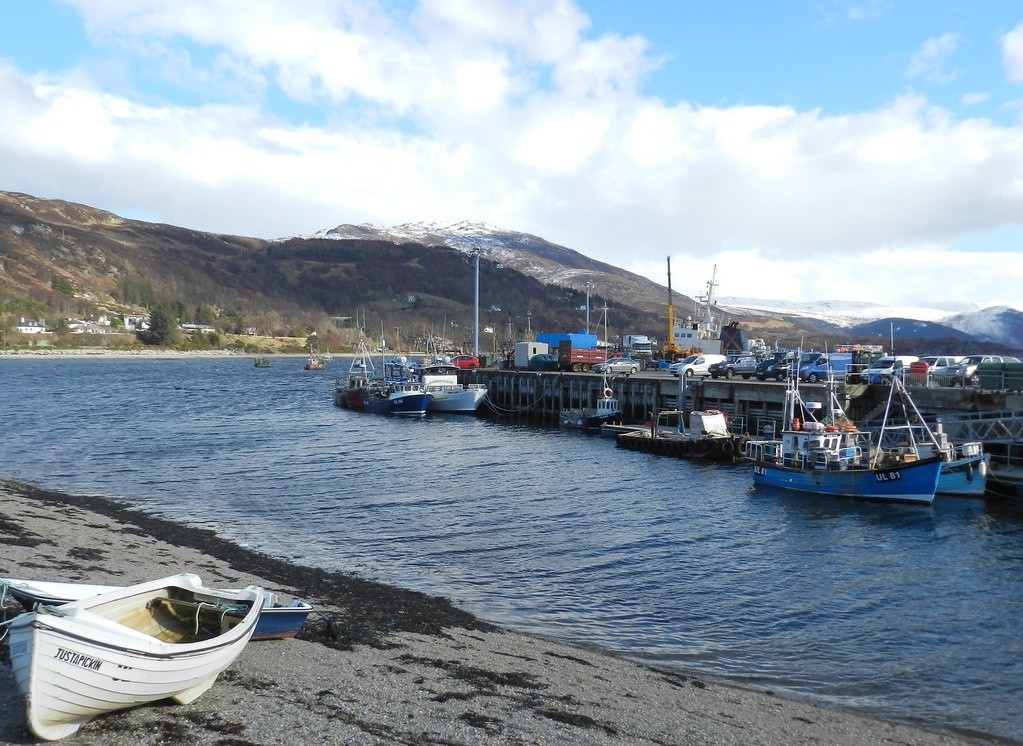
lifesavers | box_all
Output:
[825,426,857,431]
[603,387,613,398]
[706,410,721,414]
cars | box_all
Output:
[591,357,641,375]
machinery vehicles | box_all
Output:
[652,255,703,369]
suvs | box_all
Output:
[946,355,1020,390]
[708,354,759,381]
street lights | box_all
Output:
[580,282,595,335]
[467,247,488,358]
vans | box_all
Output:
[859,355,920,384]
[754,352,806,382]
[671,354,728,380]
[917,355,967,388]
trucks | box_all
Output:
[623,335,653,361]
[788,352,853,384]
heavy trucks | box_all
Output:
[559,340,623,374]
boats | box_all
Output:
[674,266,766,356]
[614,408,751,460]
[304,344,326,371]
[744,348,945,505]
[253,358,272,369]
[3,575,312,643]
[558,388,623,432]
[334,335,489,417]
[855,374,990,497]
[8,571,267,743]
